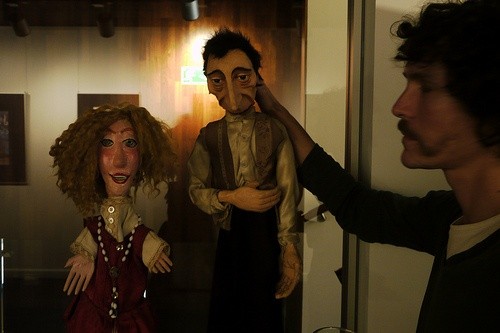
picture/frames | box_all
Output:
[78,94,139,117]
[0,94,27,186]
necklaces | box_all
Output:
[97,215,144,319]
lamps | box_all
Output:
[5,8,30,37]
[183,0,199,21]
[95,3,115,37]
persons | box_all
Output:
[48,101,181,333]
[186,28,302,333]
[255,0,500,333]
